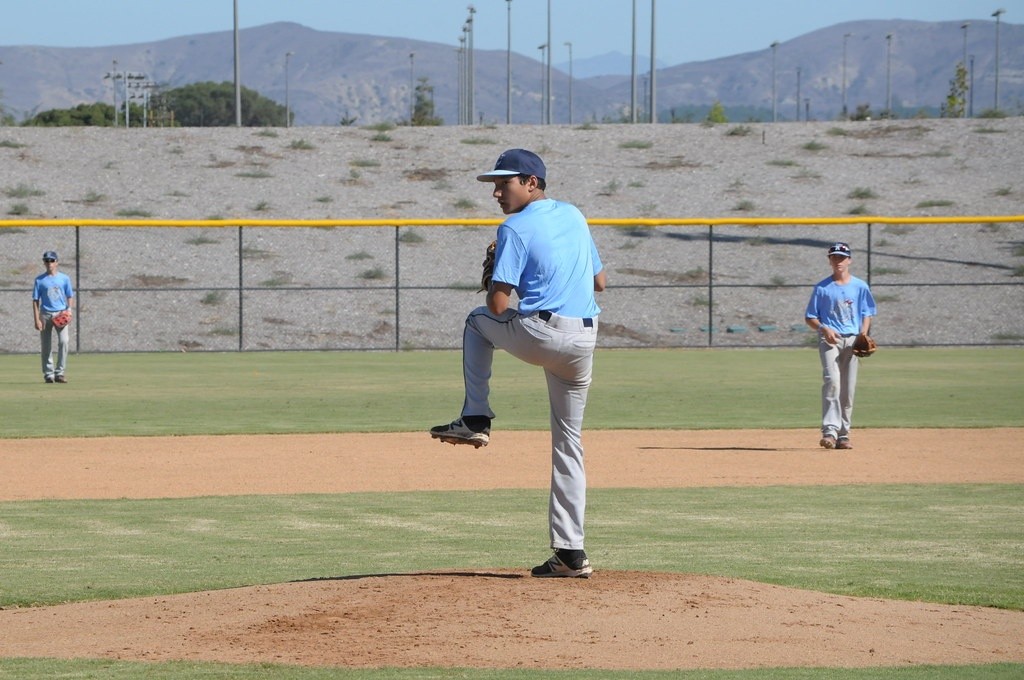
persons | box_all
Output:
[426,149,604,576]
[805,243,876,449]
[33,251,74,383]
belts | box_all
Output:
[539,310,593,327]
[838,334,853,337]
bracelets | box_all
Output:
[817,324,825,332]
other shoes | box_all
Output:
[55,375,67,383]
[836,438,853,450]
[820,435,836,448]
[46,378,53,383]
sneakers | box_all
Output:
[531,549,593,578]
[429,415,491,449]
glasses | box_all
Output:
[44,259,56,263]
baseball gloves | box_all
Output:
[52,311,72,329]
[480,239,498,291]
[852,332,877,357]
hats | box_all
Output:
[827,242,851,258]
[42,251,58,259]
[477,149,546,182]
